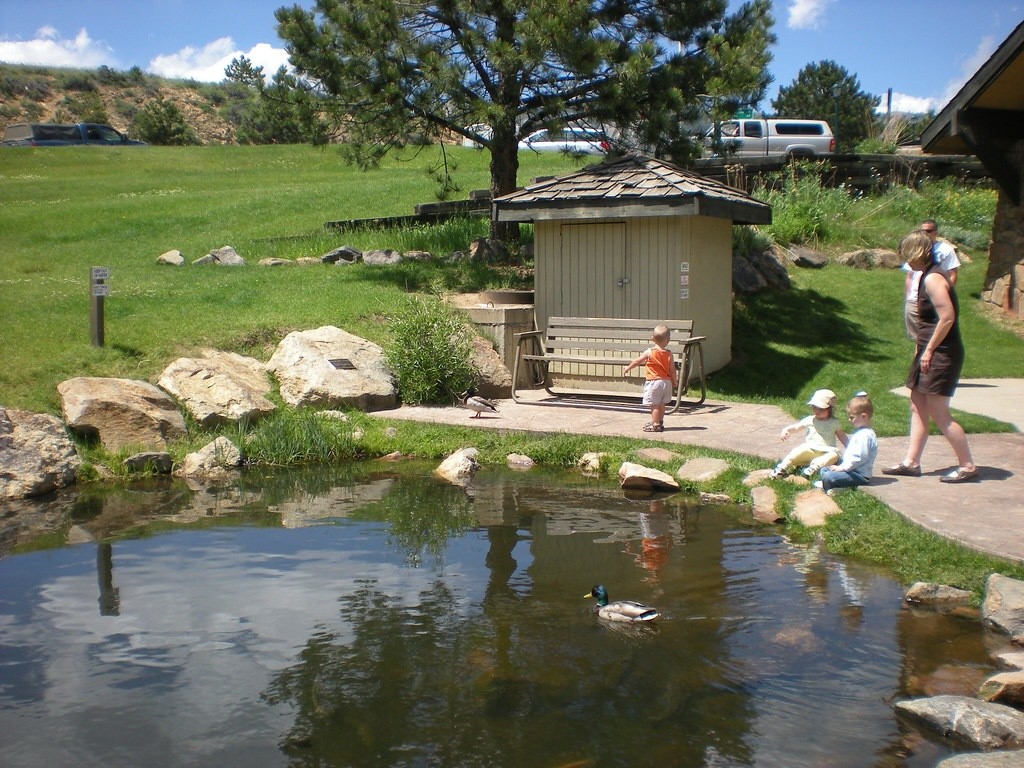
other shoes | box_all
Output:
[939,467,979,482]
[770,466,787,481]
[801,464,819,477]
[814,481,824,488]
[882,462,921,476]
[643,420,665,431]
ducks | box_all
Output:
[461,387,500,419]
[582,585,661,622]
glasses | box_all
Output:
[922,229,935,233]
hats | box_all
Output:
[807,389,837,408]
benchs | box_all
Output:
[511,316,709,414]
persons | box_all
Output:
[732,124,740,136]
[624,324,678,432]
[904,219,961,355]
[881,231,980,484]
[625,501,671,587]
[820,392,877,493]
[773,389,850,477]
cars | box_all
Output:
[518,127,610,154]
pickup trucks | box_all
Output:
[690,119,835,159]
[0,122,150,146]
